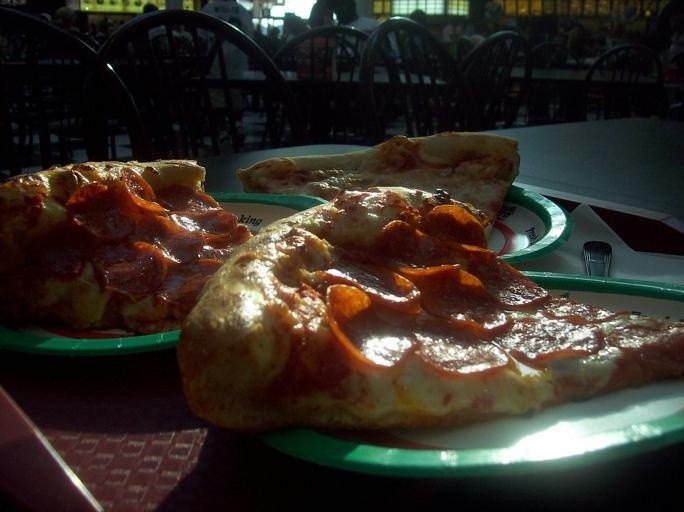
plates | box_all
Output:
[485,182,575,267]
[253,269,684,490]
[1,187,329,359]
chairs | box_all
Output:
[269,13,684,144]
[1,3,295,175]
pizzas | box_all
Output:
[175,184,684,430]
[0,157,253,337]
[234,130,521,239]
